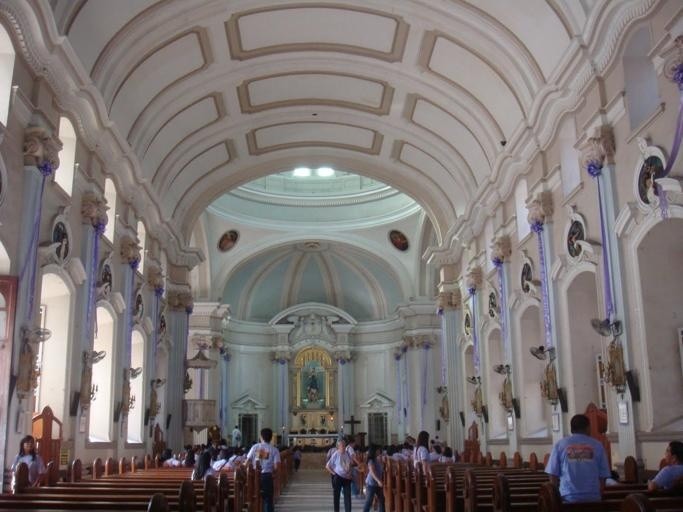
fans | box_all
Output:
[433,317,623,394]
[23,326,166,388]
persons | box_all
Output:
[9,436,46,494]
[605,470,621,487]
[546,414,611,504]
[160,426,301,512]
[325,430,456,512]
[647,440,683,494]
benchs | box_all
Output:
[0,448,293,512]
[356,448,681,512]
